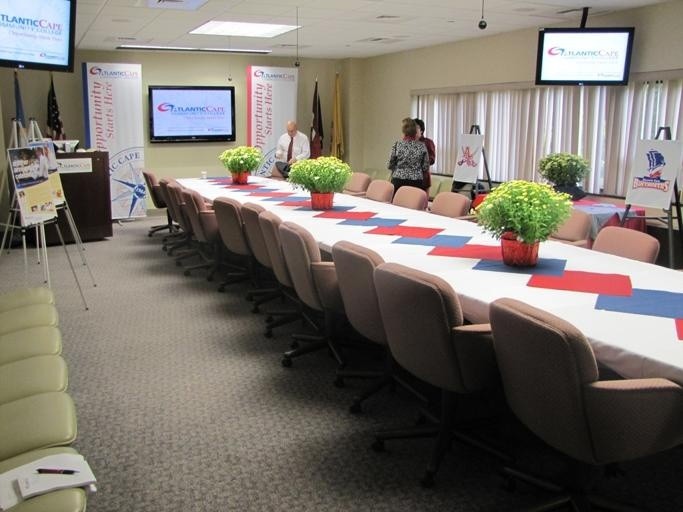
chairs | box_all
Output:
[485,292,682,510]
[330,239,395,415]
[371,263,491,493]
[138,159,682,512]
[273,159,660,263]
[141,171,349,369]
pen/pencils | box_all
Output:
[36,468,79,474]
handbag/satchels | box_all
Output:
[389,155,399,169]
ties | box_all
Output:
[288,136,294,162]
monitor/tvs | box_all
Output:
[535,28,635,86]
[148,85,235,142]
[0,0,76,72]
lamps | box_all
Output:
[294,5,300,67]
[653,126,673,140]
[476,0,487,32]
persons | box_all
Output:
[34,150,47,179]
[43,146,55,169]
[273,120,311,165]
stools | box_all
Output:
[0,285,87,512]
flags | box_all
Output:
[384,117,429,201]
[310,82,324,151]
[328,74,346,159]
[44,77,66,140]
[6,75,26,210]
[412,118,436,197]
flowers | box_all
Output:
[284,155,353,194]
[534,151,592,188]
[217,145,265,176]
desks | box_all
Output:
[6,150,114,250]
[174,176,682,386]
